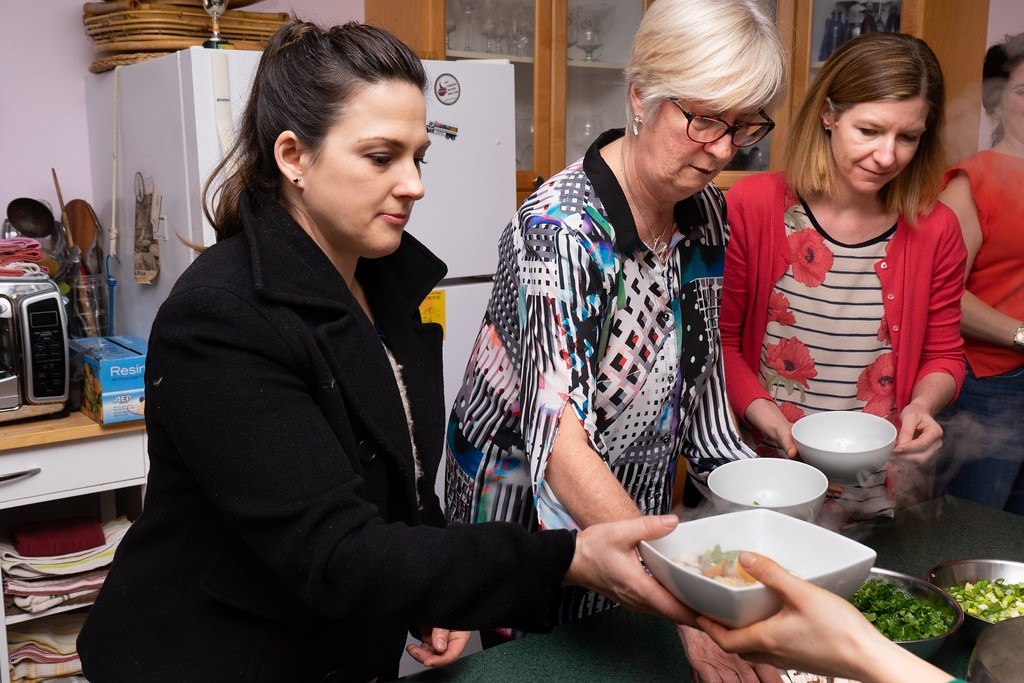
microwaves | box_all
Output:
[0,279,70,423]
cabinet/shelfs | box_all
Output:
[0,411,152,683]
[365,0,990,213]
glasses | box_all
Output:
[669,96,775,147]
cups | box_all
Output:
[573,114,603,154]
[71,274,109,339]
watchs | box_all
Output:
[1013,323,1024,346]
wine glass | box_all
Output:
[441,1,617,63]
[518,119,534,170]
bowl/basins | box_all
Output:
[706,457,829,524]
[847,567,965,660]
[636,509,878,629]
[926,558,1024,633]
[790,411,897,486]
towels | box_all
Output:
[0,236,50,278]
[6,604,94,683]
[0,512,137,617]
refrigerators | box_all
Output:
[84,45,519,675]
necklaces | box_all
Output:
[622,144,672,254]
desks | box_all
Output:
[388,491,1024,683]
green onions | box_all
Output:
[945,578,1024,623]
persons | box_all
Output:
[444,0,783,683]
[696,551,967,683]
[75,22,700,683]
[725,33,968,531]
[938,32,1024,510]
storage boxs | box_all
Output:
[72,335,149,425]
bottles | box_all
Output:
[819,9,897,61]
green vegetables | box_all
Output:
[846,575,948,641]
